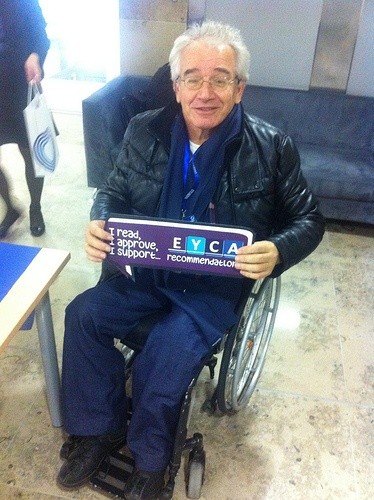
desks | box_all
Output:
[0,242,72,427]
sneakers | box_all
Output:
[55,417,129,491]
[124,468,165,500]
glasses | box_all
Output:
[181,74,238,92]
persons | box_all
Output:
[0,0,50,239]
[57,20,327,500]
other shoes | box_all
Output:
[0,206,27,236]
[29,204,45,236]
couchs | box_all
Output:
[82,75,374,226]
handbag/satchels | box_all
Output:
[22,79,62,178]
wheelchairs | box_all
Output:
[59,273,282,500]
